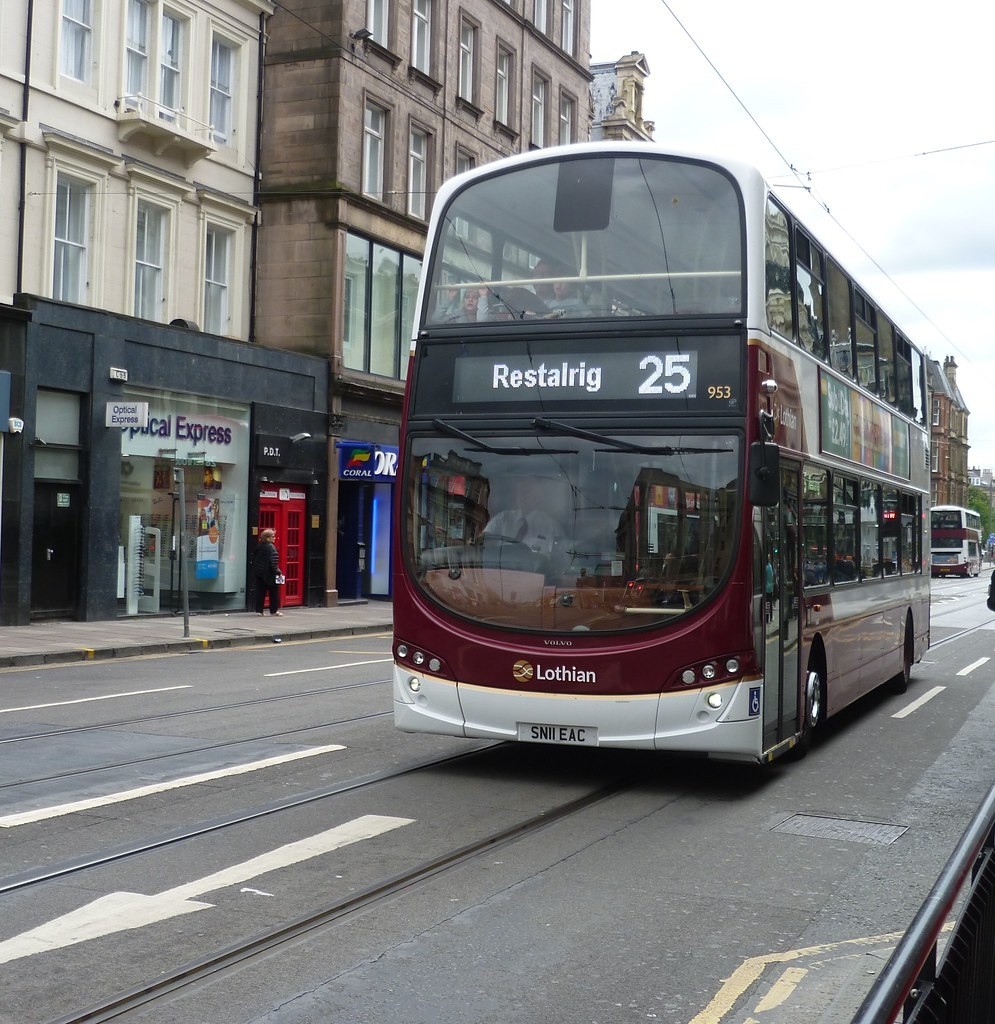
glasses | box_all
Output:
[268,535,275,538]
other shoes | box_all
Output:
[256,612,266,617]
[270,611,283,617]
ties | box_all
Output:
[514,518,529,543]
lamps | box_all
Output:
[350,26,374,39]
[288,431,313,444]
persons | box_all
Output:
[252,529,284,616]
[766,554,773,622]
[468,481,567,557]
[431,281,490,323]
[527,258,595,318]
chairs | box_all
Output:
[832,557,899,580]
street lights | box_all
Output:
[989,478,995,541]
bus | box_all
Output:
[389,139,933,763]
[929,504,984,577]
